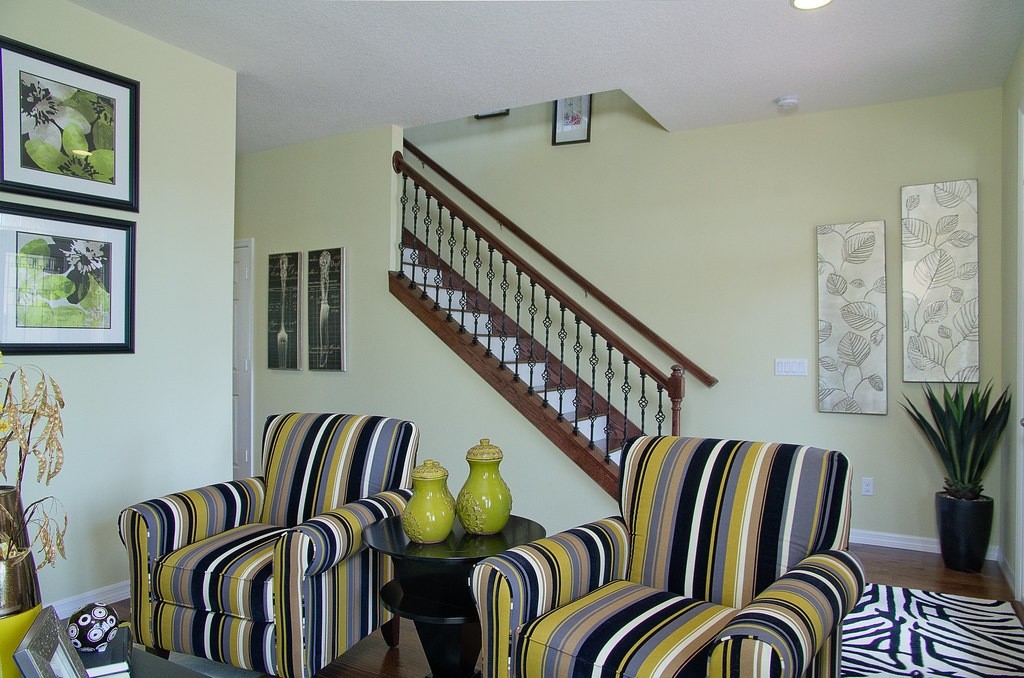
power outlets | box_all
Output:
[861,477,873,496]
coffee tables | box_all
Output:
[131,648,212,678]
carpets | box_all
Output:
[840,582,1024,678]
[132,643,268,678]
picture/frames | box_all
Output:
[0,200,135,355]
[13,606,89,678]
[307,247,346,373]
[474,109,509,119]
[267,251,303,371]
[0,35,141,213]
[551,94,592,146]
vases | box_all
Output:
[0,486,42,617]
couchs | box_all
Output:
[466,435,865,678]
[117,413,420,678]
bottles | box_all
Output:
[456,438,512,534]
[400,460,455,544]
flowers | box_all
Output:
[0,351,68,570]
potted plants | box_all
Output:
[898,375,1012,574]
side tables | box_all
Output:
[361,513,547,678]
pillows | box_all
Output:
[0,602,43,678]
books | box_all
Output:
[12,605,133,678]
